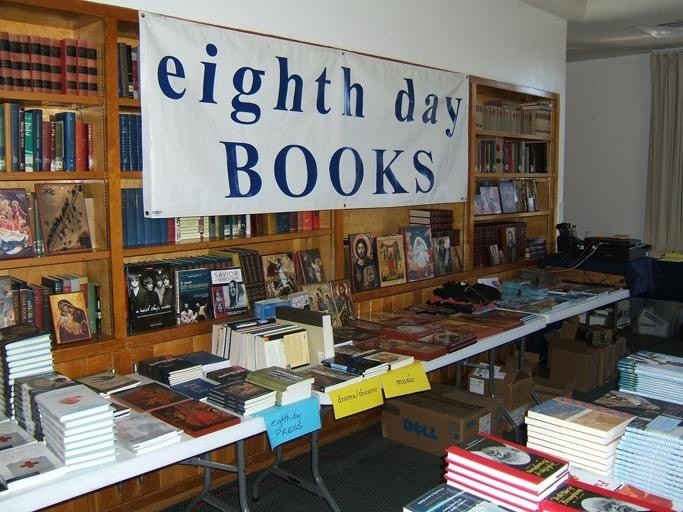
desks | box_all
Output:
[0,279,630,510]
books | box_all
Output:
[475,266,623,314]
[2,32,99,98]
[122,188,357,370]
[117,43,143,171]
[307,302,537,394]
[1,102,95,259]
[401,390,683,512]
[616,350,683,405]
[344,210,462,292]
[81,350,315,455]
[476,100,554,269]
[2,273,116,494]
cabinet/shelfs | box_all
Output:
[0,1,557,510]
